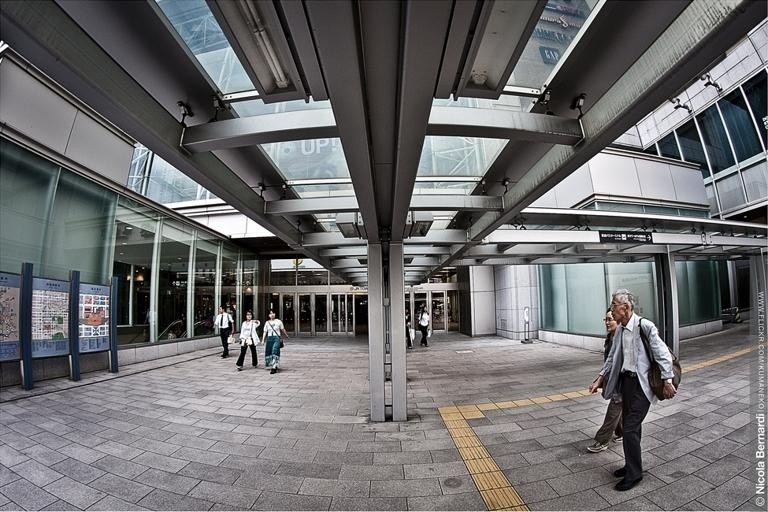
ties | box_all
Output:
[220,315,223,326]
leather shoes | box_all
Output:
[615,476,642,491]
[613,467,627,477]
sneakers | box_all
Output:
[270,367,279,374]
[613,435,625,443]
[252,364,256,368]
[237,366,243,369]
[587,441,608,453]
[221,352,229,357]
[420,343,428,347]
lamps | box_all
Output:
[569,93,586,119]
[177,99,194,127]
[451,218,457,229]
[207,96,226,122]
[313,220,317,233]
[258,182,266,200]
[700,73,722,92]
[280,182,289,200]
[667,95,693,113]
[481,178,488,196]
[469,217,473,227]
[540,89,559,116]
[295,219,302,232]
[246,281,249,289]
[502,179,509,194]
[237,0,290,89]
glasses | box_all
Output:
[609,303,627,312]
[603,319,614,322]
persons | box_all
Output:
[588,289,678,491]
[260,309,290,374]
[168,330,177,339]
[235,310,261,370]
[586,308,624,453]
[204,305,235,359]
[405,305,431,349]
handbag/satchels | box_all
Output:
[278,339,284,348]
[649,347,680,401]
[228,337,235,344]
[246,336,258,346]
